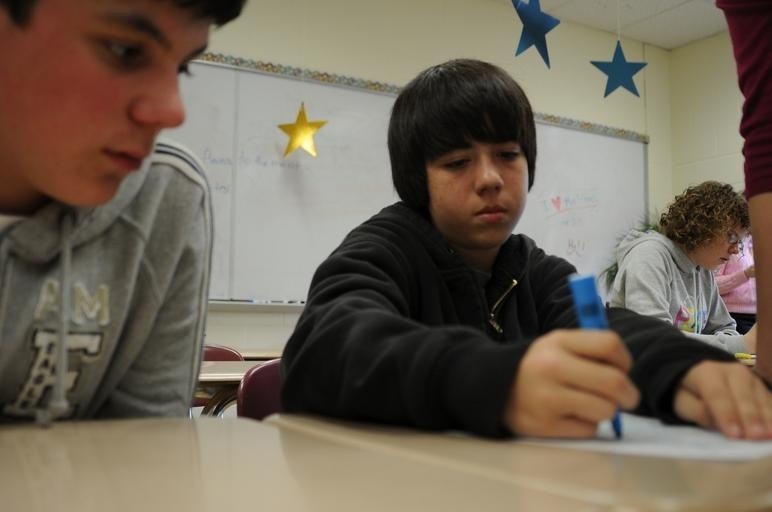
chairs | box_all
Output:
[190,345,243,406]
[235,357,283,421]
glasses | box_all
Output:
[727,231,744,252]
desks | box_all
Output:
[196,360,267,415]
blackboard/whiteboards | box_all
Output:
[155,54,651,306]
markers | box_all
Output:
[567,274,623,440]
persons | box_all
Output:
[278,60,772,443]
[607,180,757,357]
[0,0,248,425]
[712,235,757,335]
[714,0,772,393]
